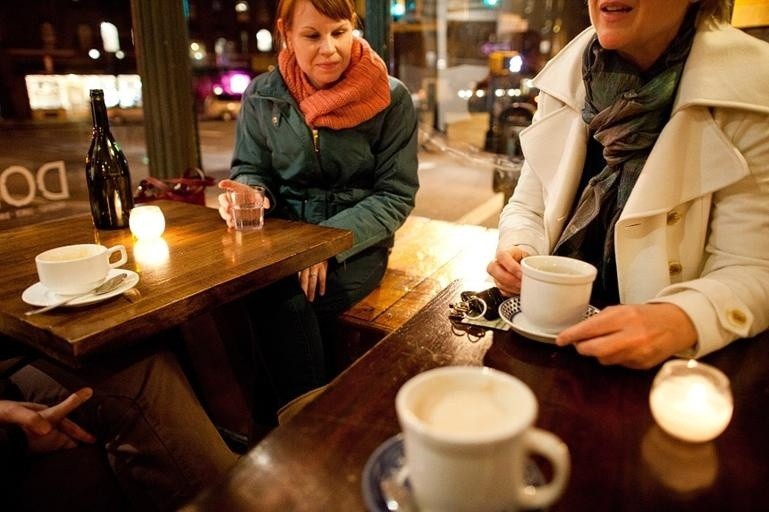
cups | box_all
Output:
[35,244,127,295]
[226,185,265,231]
[518,255,596,330]
[395,367,570,512]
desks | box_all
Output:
[196,271,769,512]
[0,198,354,482]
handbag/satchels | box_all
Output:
[132,167,217,205]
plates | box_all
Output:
[499,297,602,344]
[21,269,140,307]
[359,428,549,512]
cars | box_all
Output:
[204,92,242,121]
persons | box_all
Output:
[0,343,240,511]
[218,0,418,432]
[487,0,768,370]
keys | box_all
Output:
[451,288,506,322]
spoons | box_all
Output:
[23,272,128,316]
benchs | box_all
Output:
[342,214,499,336]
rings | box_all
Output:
[309,272,318,277]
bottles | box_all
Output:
[646,359,735,446]
[85,89,135,230]
